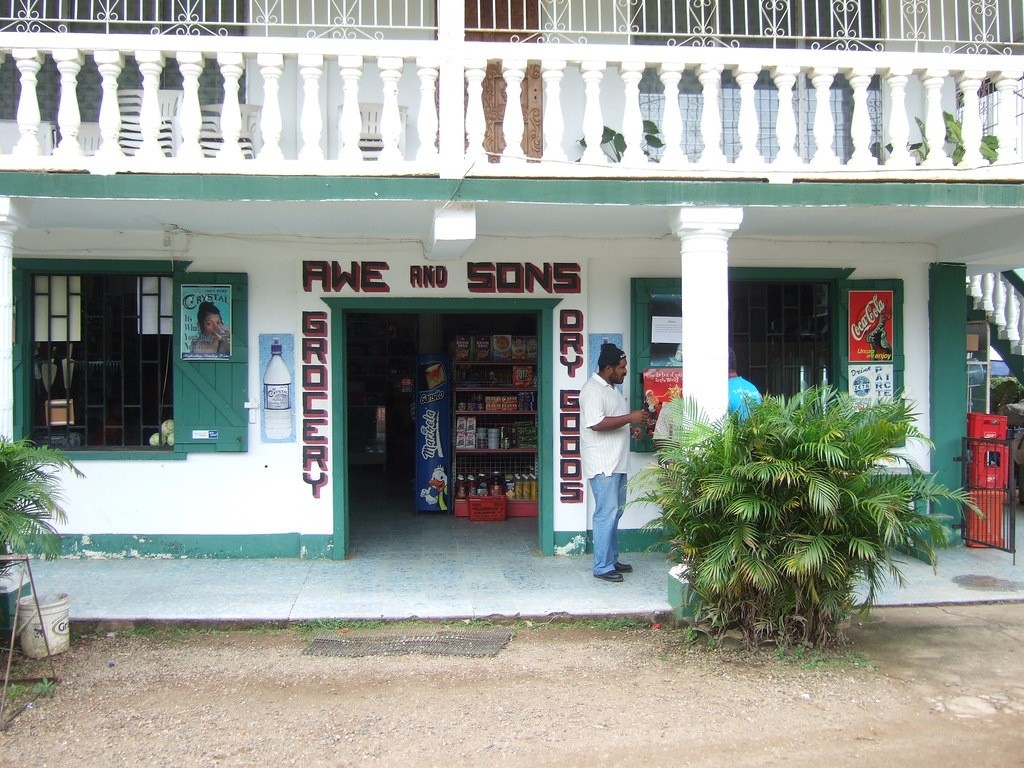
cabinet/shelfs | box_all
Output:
[453,360,538,517]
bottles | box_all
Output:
[595,339,609,373]
[456,473,476,499]
[456,368,461,380]
[506,473,538,498]
[489,472,504,496]
[462,368,466,379]
[477,474,489,496]
[264,338,292,439]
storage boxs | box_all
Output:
[668,564,700,618]
[966,413,1007,445]
[513,365,532,388]
[485,396,517,411]
[968,442,1009,489]
[468,495,507,520]
[475,335,491,360]
[492,335,511,360]
[512,336,538,360]
[456,416,476,449]
[0,575,31,634]
[456,335,470,361]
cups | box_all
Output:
[214,323,230,343]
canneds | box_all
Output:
[517,391,537,412]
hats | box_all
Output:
[597,343,626,366]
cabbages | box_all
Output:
[149,419,174,447]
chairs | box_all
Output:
[76,122,103,155]
[338,103,408,162]
[116,89,263,158]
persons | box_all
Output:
[192,301,230,353]
[728,347,764,427]
[652,402,690,494]
[579,342,649,581]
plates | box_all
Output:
[512,344,525,355]
[494,336,511,351]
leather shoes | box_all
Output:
[593,571,623,582]
[614,562,632,572]
